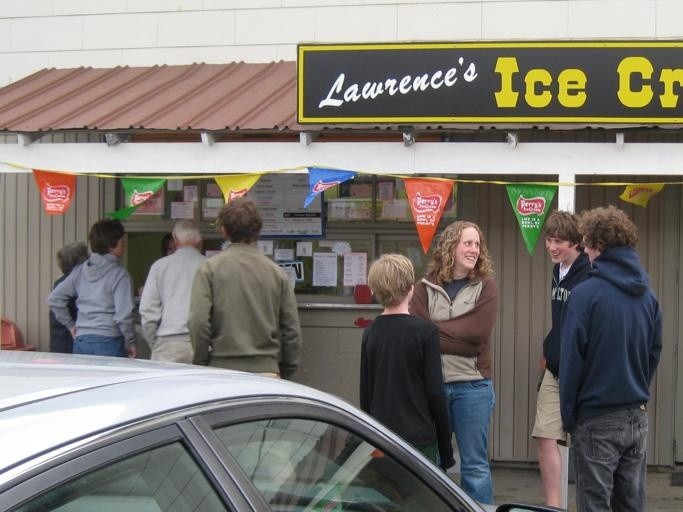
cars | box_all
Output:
[0,342,569,512]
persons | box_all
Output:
[44,217,138,362]
[358,252,457,472]
[555,203,663,511]
[185,196,301,381]
[138,217,208,366]
[138,232,176,296]
[45,241,90,353]
[406,219,499,505]
[528,210,590,510]
